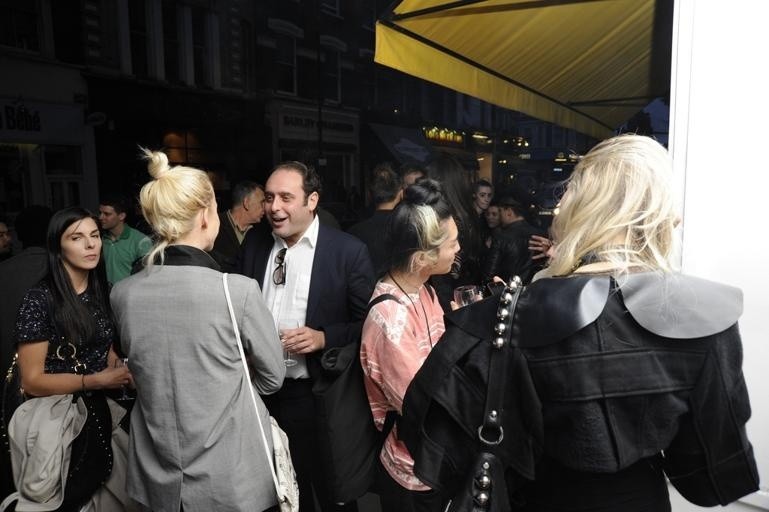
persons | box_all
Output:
[1,142,558,511]
[395,133,764,511]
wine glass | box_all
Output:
[276,317,301,368]
[113,356,137,404]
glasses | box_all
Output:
[273,248,287,286]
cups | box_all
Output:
[454,284,479,307]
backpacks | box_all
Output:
[313,283,434,508]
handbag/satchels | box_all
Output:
[443,274,525,511]
[0,342,113,512]
[266,413,302,512]
